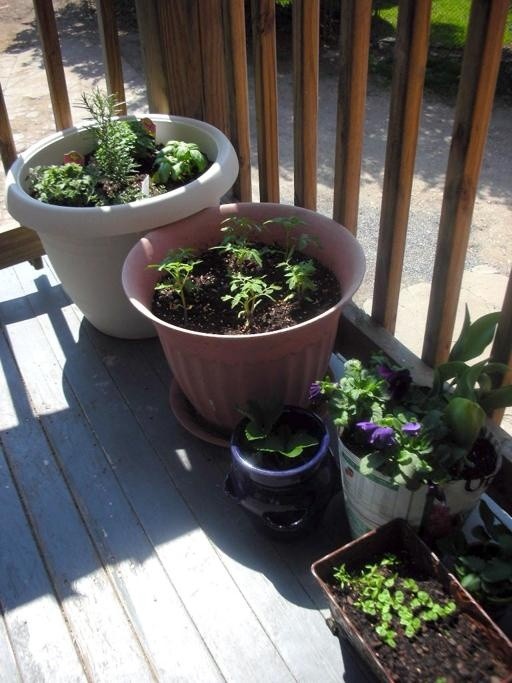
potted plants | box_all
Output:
[222,400,340,538]
[310,514,512,683]
[4,86,369,448]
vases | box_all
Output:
[333,417,505,540]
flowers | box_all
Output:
[307,306,512,497]
